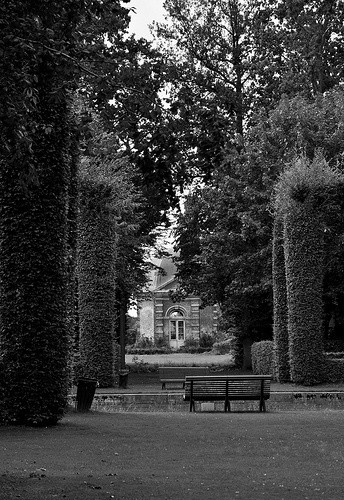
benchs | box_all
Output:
[158,366,211,389]
[181,373,273,413]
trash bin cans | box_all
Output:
[75,376,98,412]
[118,372,129,388]
